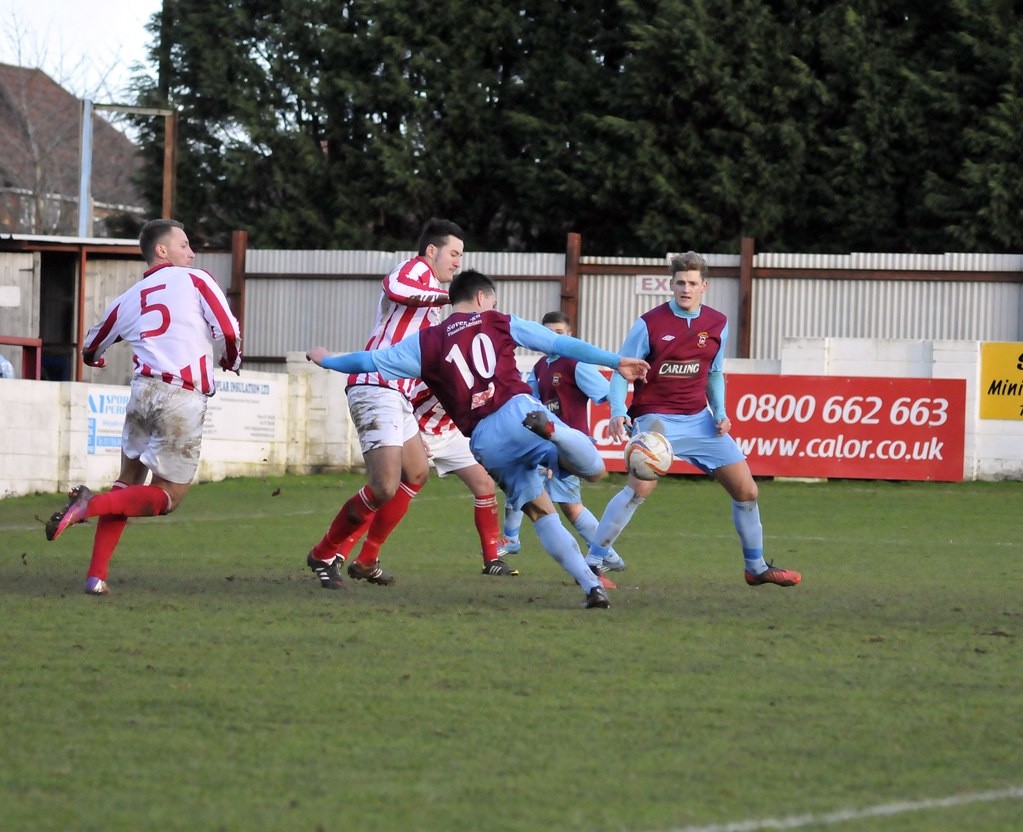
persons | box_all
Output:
[496,312,624,572]
[46,218,241,592]
[306,269,651,607]
[576,252,801,588]
[306,219,466,590]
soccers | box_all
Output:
[623,432,673,481]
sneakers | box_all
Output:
[575,565,618,589]
[521,411,555,438]
[496,538,521,556]
[582,586,610,609]
[348,557,395,586]
[307,548,344,589]
[745,558,800,587]
[480,558,519,576]
[84,577,109,594]
[47,486,94,541]
[599,556,625,573]
